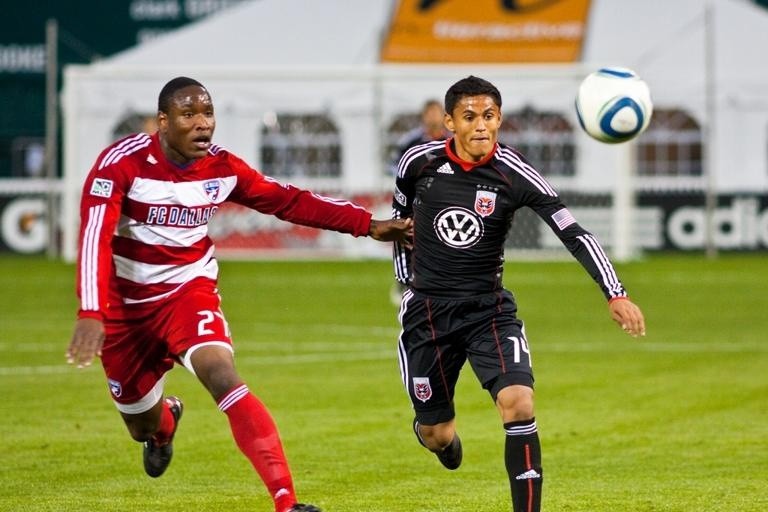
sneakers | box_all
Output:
[143,396,182,477]
[414,415,462,469]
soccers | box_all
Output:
[574,68,651,141]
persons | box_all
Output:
[392,101,448,167]
[393,85,647,512]
[68,76,414,512]
